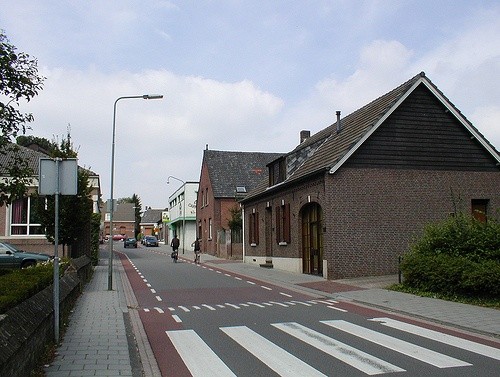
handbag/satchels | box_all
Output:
[171,252,175,258]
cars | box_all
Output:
[140,235,152,246]
[146,236,159,247]
[105,233,127,241]
[123,238,138,248]
[0,240,51,274]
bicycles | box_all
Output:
[171,245,179,263]
[192,246,201,264]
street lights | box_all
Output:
[167,176,185,254]
[108,95,164,290]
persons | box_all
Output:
[191,238,200,259]
[171,235,180,255]
